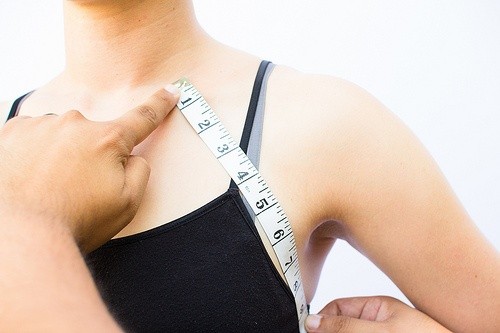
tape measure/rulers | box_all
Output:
[167,79,309,332]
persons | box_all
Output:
[1,84,454,332]
[1,0,499,332]
